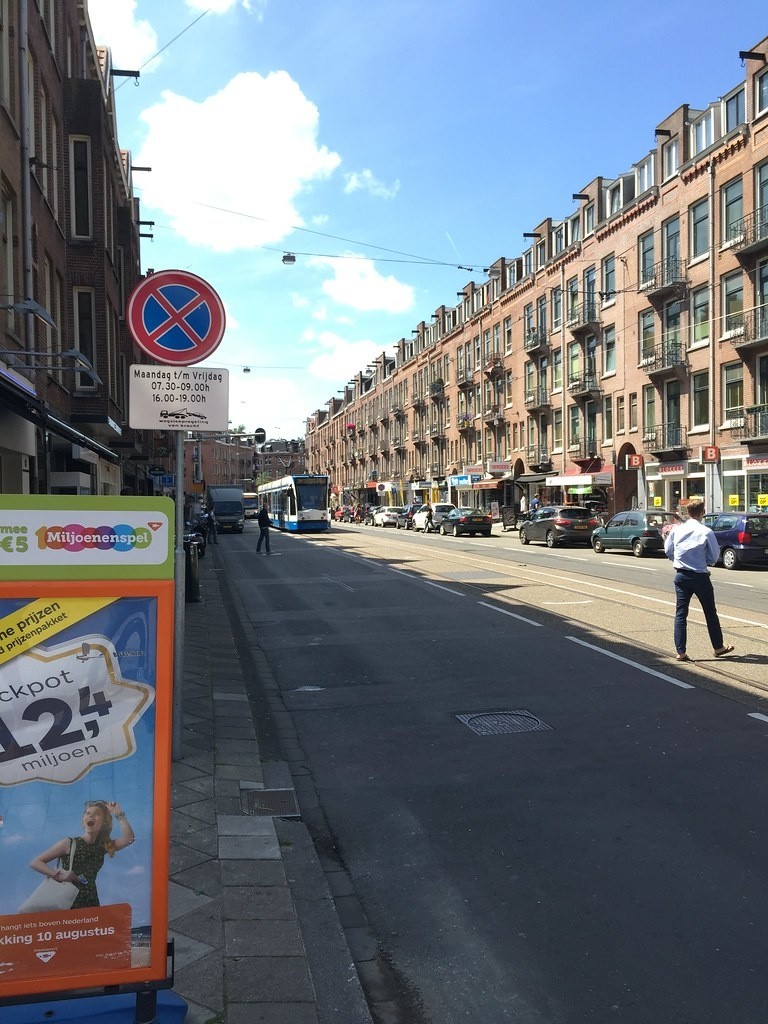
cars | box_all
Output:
[519,505,602,548]
[591,509,685,557]
[702,511,768,570]
[331,501,457,533]
[439,507,493,537]
[174,521,206,557]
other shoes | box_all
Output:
[267,550,274,555]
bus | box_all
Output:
[257,474,331,532]
[244,492,258,518]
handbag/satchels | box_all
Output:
[207,512,215,525]
[18,836,80,913]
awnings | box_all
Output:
[515,472,612,486]
[474,479,506,489]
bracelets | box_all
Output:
[115,811,125,820]
[54,871,62,881]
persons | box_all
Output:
[664,499,734,661]
[520,493,540,521]
[184,501,193,531]
[207,505,220,544]
[256,503,274,554]
[120,489,130,496]
[421,503,437,533]
[30,800,136,909]
[330,503,371,525]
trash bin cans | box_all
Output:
[182,533,202,603]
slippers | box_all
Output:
[714,645,733,656]
[676,653,687,661]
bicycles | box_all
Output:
[355,515,360,527]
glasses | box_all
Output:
[85,799,107,808]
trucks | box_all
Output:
[206,483,246,533]
